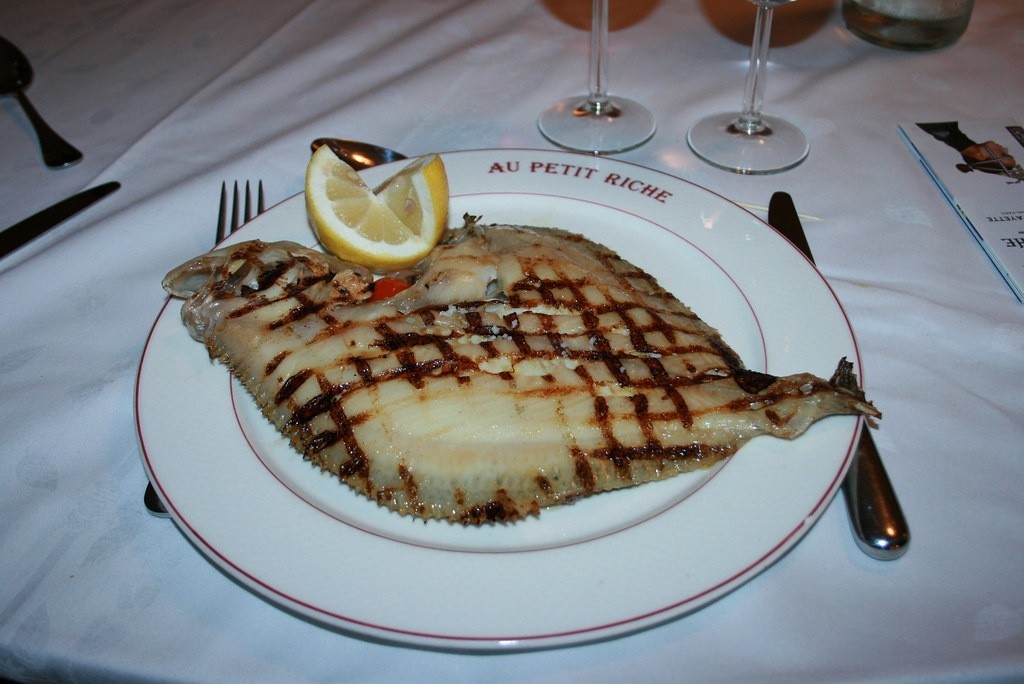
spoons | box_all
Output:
[0,35,83,171]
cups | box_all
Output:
[694,0,832,48]
[534,0,660,33]
[841,0,975,52]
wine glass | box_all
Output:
[685,0,811,175]
[537,0,657,155]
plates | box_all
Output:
[133,147,866,652]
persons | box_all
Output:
[915,121,1016,176]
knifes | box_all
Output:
[0,181,121,262]
[769,191,911,562]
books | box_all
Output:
[896,117,1024,306]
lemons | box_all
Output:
[305,142,450,275]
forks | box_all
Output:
[143,179,264,516]
